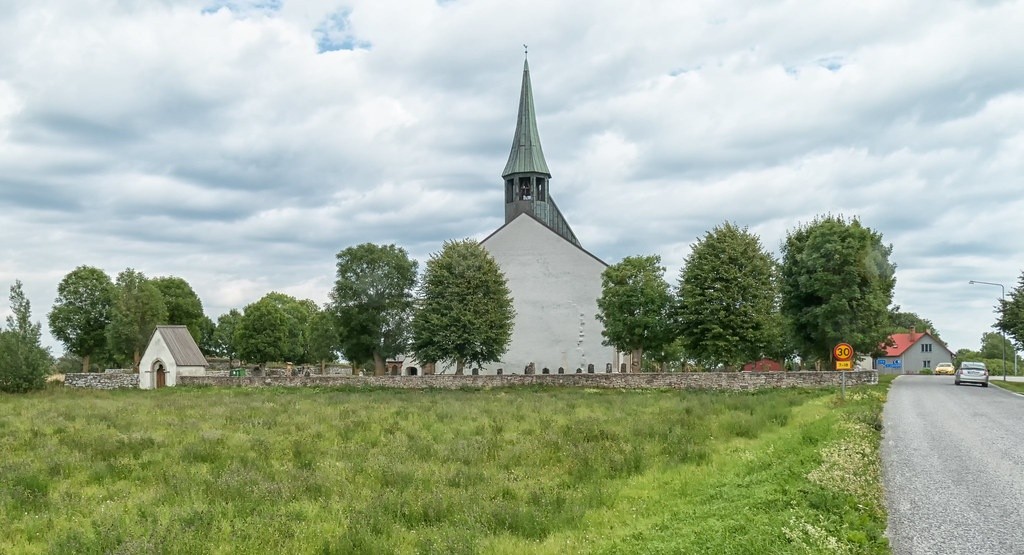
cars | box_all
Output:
[955,361,990,387]
[935,363,955,375]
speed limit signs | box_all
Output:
[833,343,854,361]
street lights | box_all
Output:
[969,280,1006,380]
